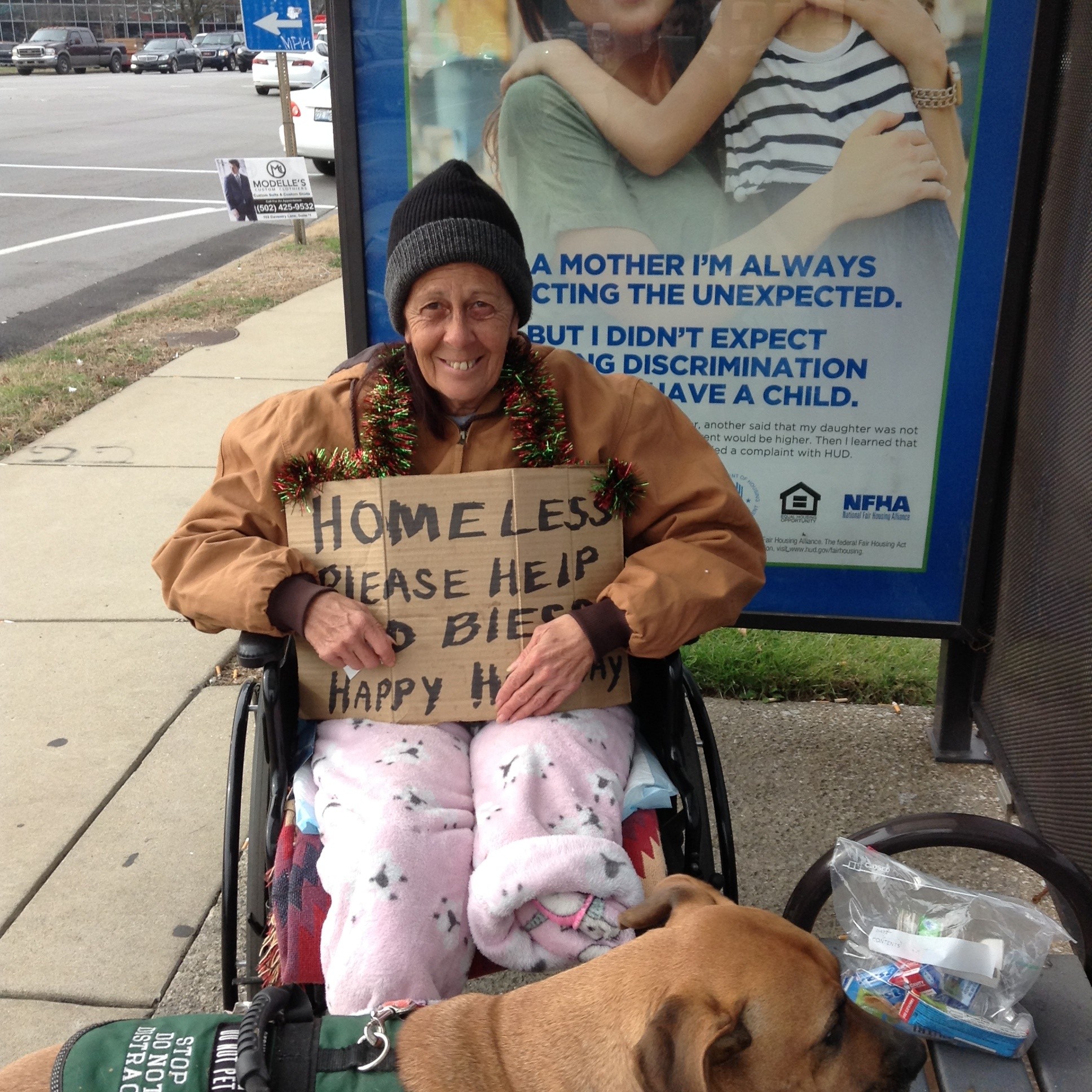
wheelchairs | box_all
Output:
[221,629,738,1013]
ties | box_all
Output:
[236,175,246,204]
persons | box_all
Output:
[151,160,766,1016]
[484,0,969,377]
[223,160,257,221]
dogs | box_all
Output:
[0,873,927,1091]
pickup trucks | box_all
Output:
[11,27,128,76]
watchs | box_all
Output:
[911,62,963,108]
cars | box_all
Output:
[0,40,20,61]
[191,15,405,95]
[278,60,407,176]
[130,39,202,74]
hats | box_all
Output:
[385,160,534,336]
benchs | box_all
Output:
[782,813,1092,1092]
